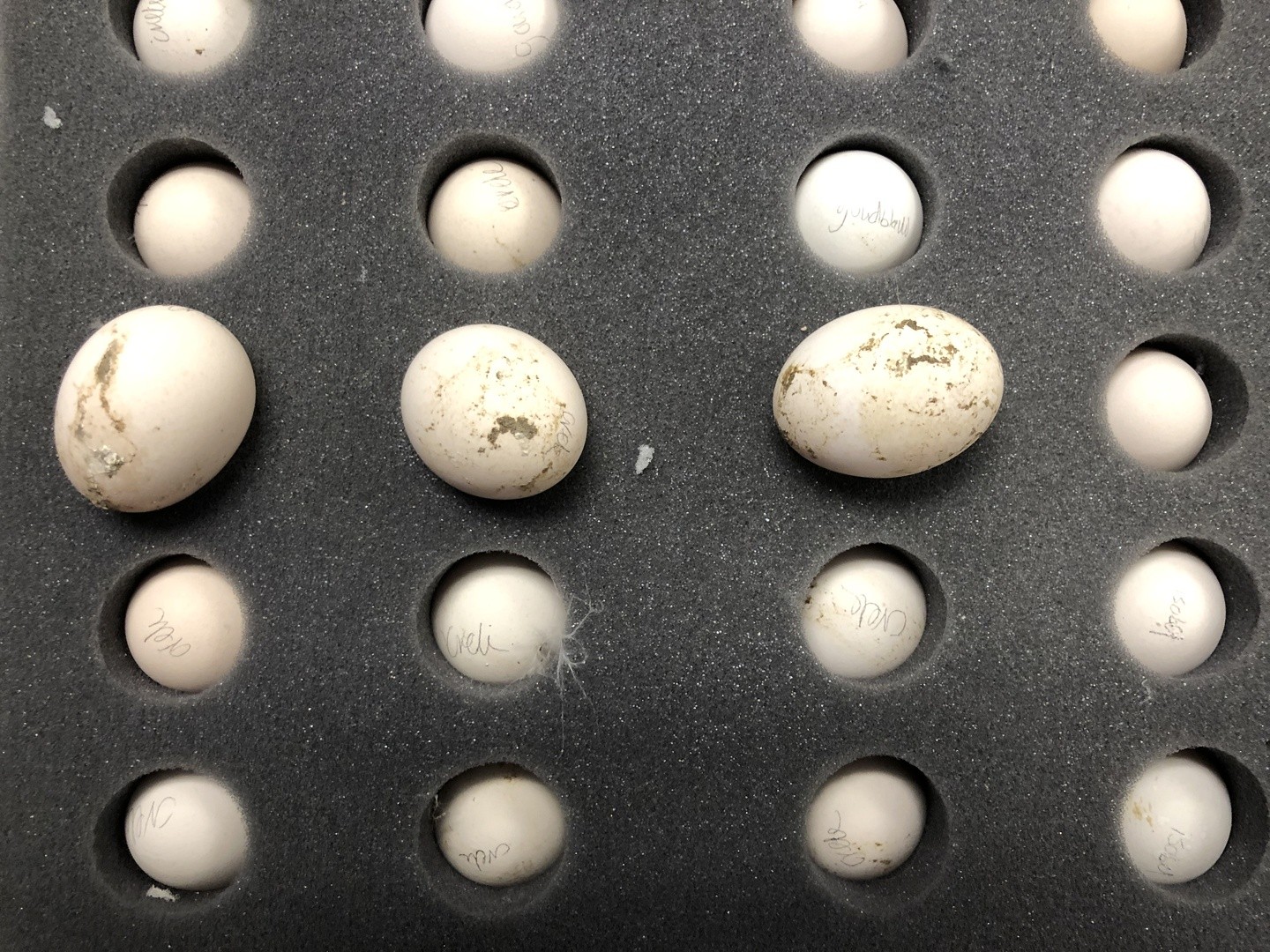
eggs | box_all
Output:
[53,0,1233,889]
